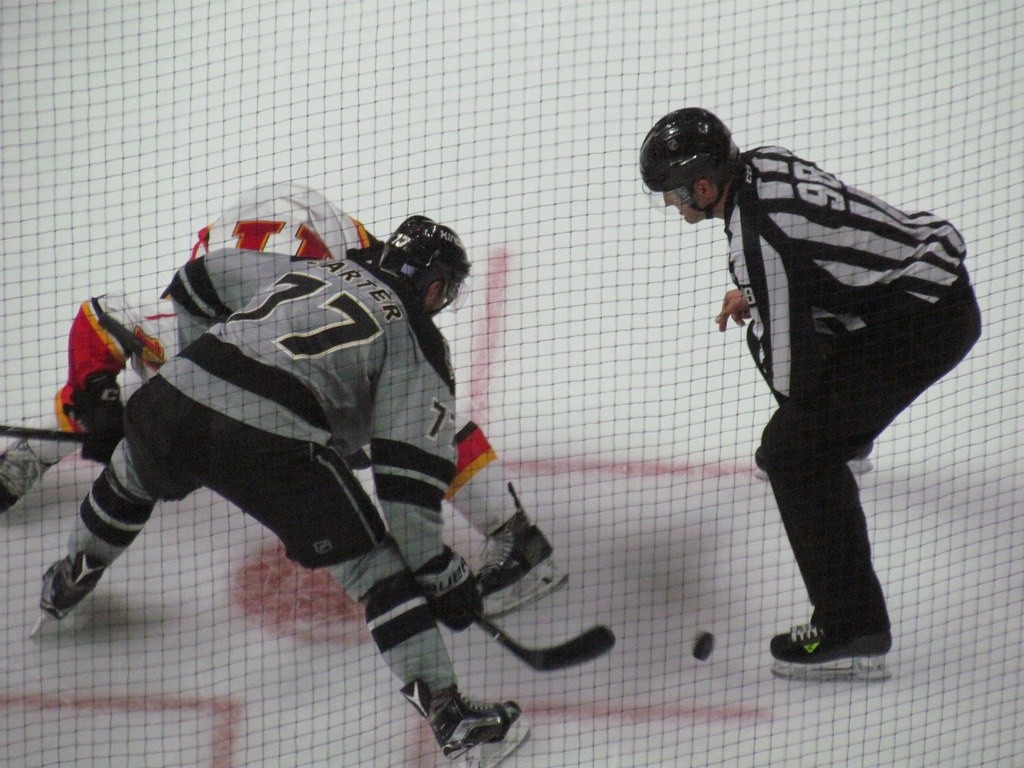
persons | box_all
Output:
[29,215,534,768]
[639,108,983,683]
[0,182,567,622]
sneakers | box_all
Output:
[28,551,106,636]
[0,438,63,513]
[400,679,529,768]
[472,482,569,618]
[755,442,874,480]
[769,623,892,683]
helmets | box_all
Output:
[638,107,731,192]
[386,215,469,281]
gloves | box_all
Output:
[77,373,128,463]
[413,544,482,631]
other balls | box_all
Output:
[692,631,713,661]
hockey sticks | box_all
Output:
[2,421,374,474]
[97,309,619,674]
